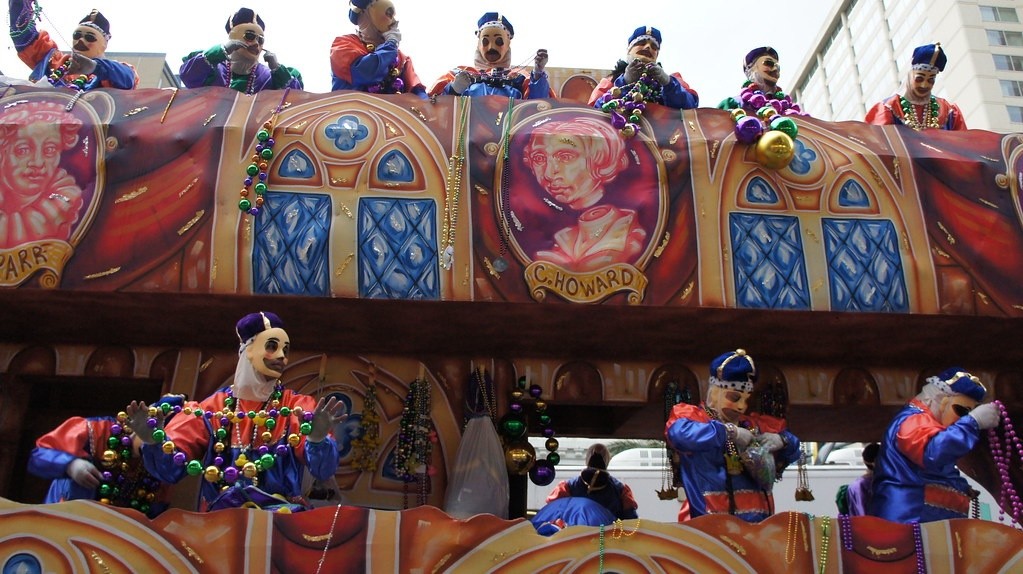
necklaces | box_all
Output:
[352,375,433,510]
[475,365,561,486]
[603,65,663,137]
[785,510,853,574]
[900,96,940,129]
[99,381,314,512]
[440,51,538,270]
[8,0,88,110]
[226,60,258,92]
[912,521,925,574]
[740,84,810,116]
[988,400,1023,529]
[599,519,641,574]
[359,32,404,94]
[703,406,761,458]
[239,88,290,214]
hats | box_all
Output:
[348,0,377,26]
[79,9,111,41]
[911,42,947,73]
[148,394,188,408]
[225,8,265,34]
[477,12,514,40]
[742,46,779,73]
[708,348,757,391]
[236,311,283,357]
[627,26,661,53]
[925,368,988,402]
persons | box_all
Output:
[29,393,188,519]
[180,8,304,93]
[588,26,699,109]
[8,0,139,90]
[546,444,639,520]
[717,46,780,110]
[124,312,347,513]
[531,497,617,537]
[330,0,426,99]
[848,444,882,516]
[865,43,968,130]
[665,350,800,524]
[869,368,1001,522]
[428,12,555,99]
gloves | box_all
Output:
[733,425,753,448]
[532,49,548,75]
[452,70,472,94]
[379,21,401,42]
[262,49,279,70]
[67,53,97,76]
[224,38,249,55]
[756,432,784,453]
[968,402,1001,429]
[649,62,670,87]
[623,59,645,84]
